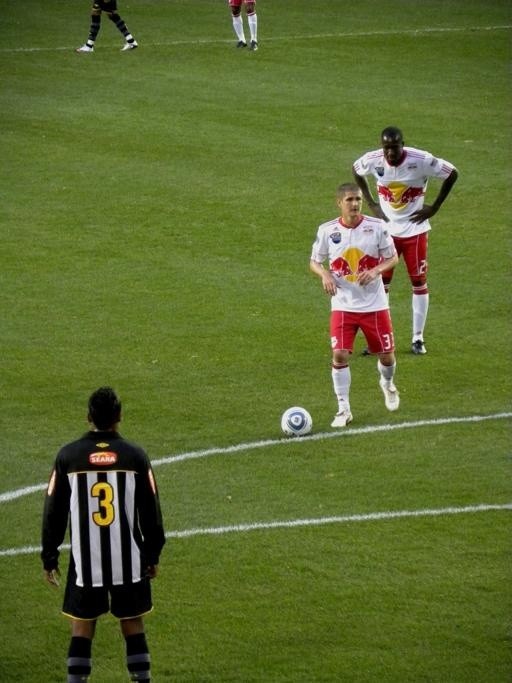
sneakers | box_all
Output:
[410,340,427,354]
[120,38,138,51]
[75,43,94,53]
[236,41,247,48]
[378,380,399,413]
[330,409,354,428]
[250,40,259,52]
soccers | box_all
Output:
[280,406,314,436]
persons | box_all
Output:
[40,385,167,683]
[73,0,139,53]
[308,181,400,429]
[228,0,260,52]
[351,125,460,355]
